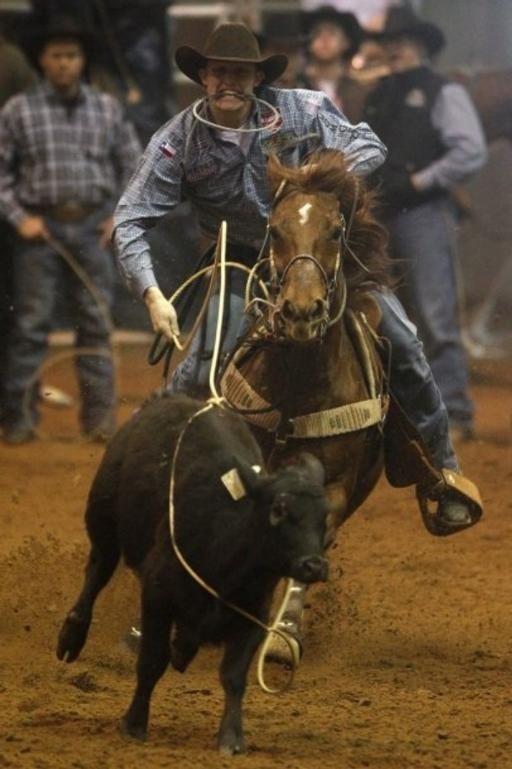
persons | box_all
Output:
[110,21,476,533]
[357,0,493,443]
[0,10,142,445]
[285,3,365,124]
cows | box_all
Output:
[56,388,335,754]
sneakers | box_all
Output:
[437,465,473,526]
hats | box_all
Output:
[295,4,362,56]
[20,15,94,70]
[174,23,288,86]
[361,5,446,57]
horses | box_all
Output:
[218,150,389,667]
